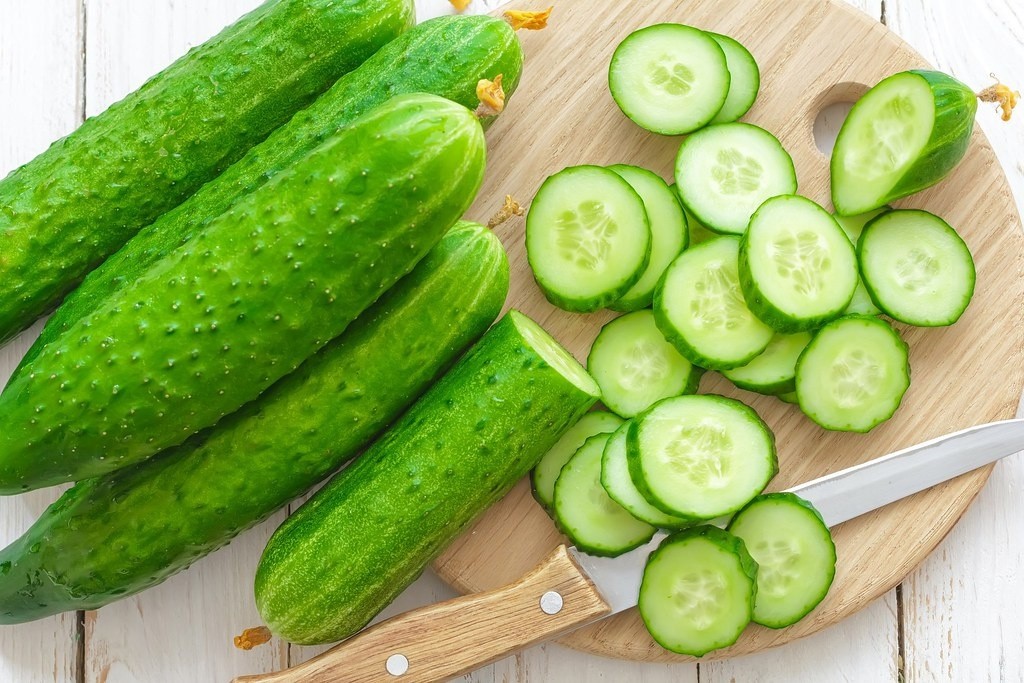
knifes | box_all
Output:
[227,418,1024,682]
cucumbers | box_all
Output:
[0,1,1021,658]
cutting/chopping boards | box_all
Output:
[427,0,1023,664]
